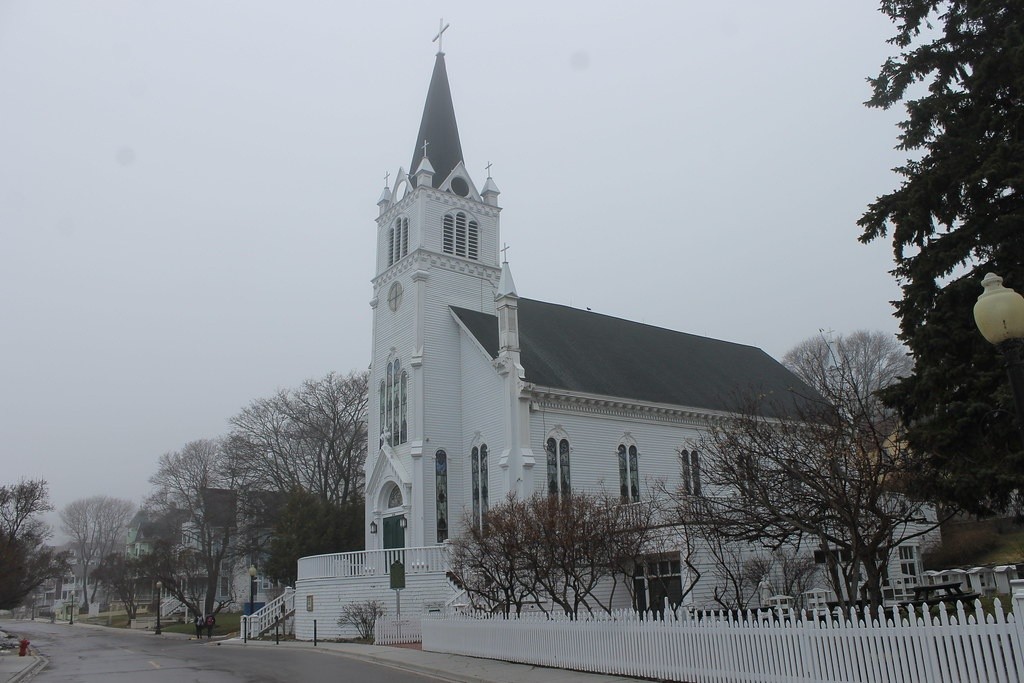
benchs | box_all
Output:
[914,594,982,616]
[898,597,957,615]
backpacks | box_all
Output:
[197,616,203,625]
[208,616,212,625]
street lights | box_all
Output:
[973,271,1024,435]
[155,580,162,635]
[69,589,75,624]
[32,595,35,620]
[249,565,256,614]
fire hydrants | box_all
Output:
[18,637,30,657]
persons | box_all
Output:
[759,573,774,606]
[205,613,215,640]
[194,612,206,639]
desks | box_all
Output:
[926,581,965,597]
